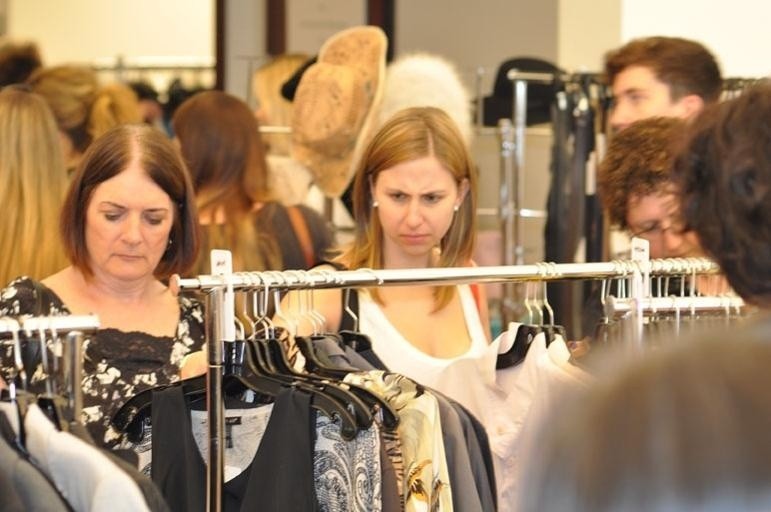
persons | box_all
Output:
[1,32,770,510]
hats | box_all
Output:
[289,25,389,201]
[472,58,566,113]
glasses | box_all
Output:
[632,216,691,243]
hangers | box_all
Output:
[0,314,66,458]
[497,260,742,370]
[112,265,400,440]
